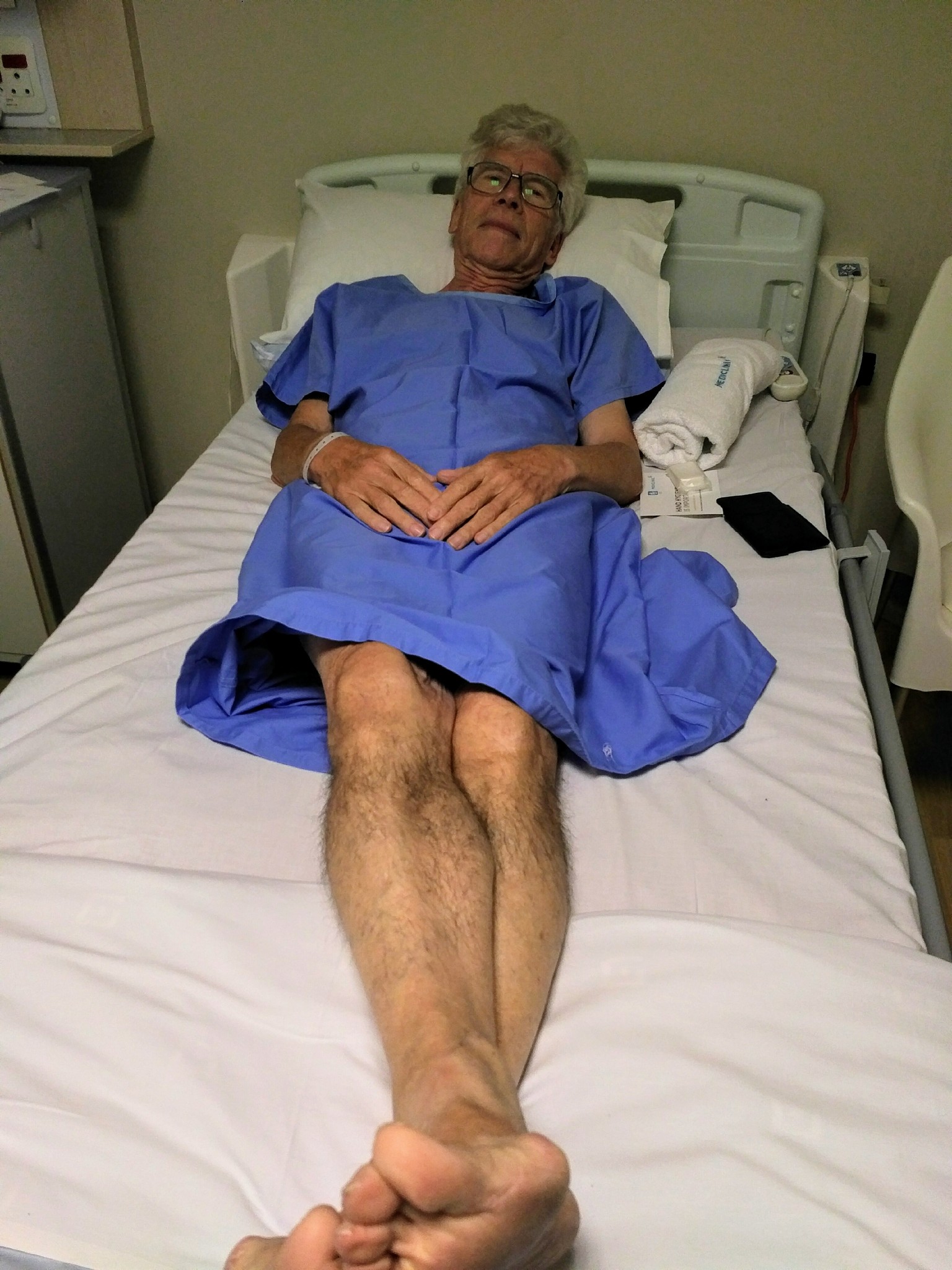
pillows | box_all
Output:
[252,178,677,371]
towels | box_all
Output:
[633,335,788,471]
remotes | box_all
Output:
[769,352,809,401]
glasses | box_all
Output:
[466,161,564,216]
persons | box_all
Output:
[175,105,776,1270]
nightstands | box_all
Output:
[0,165,152,667]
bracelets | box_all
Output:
[303,432,348,489]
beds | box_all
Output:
[0,154,952,1270]
